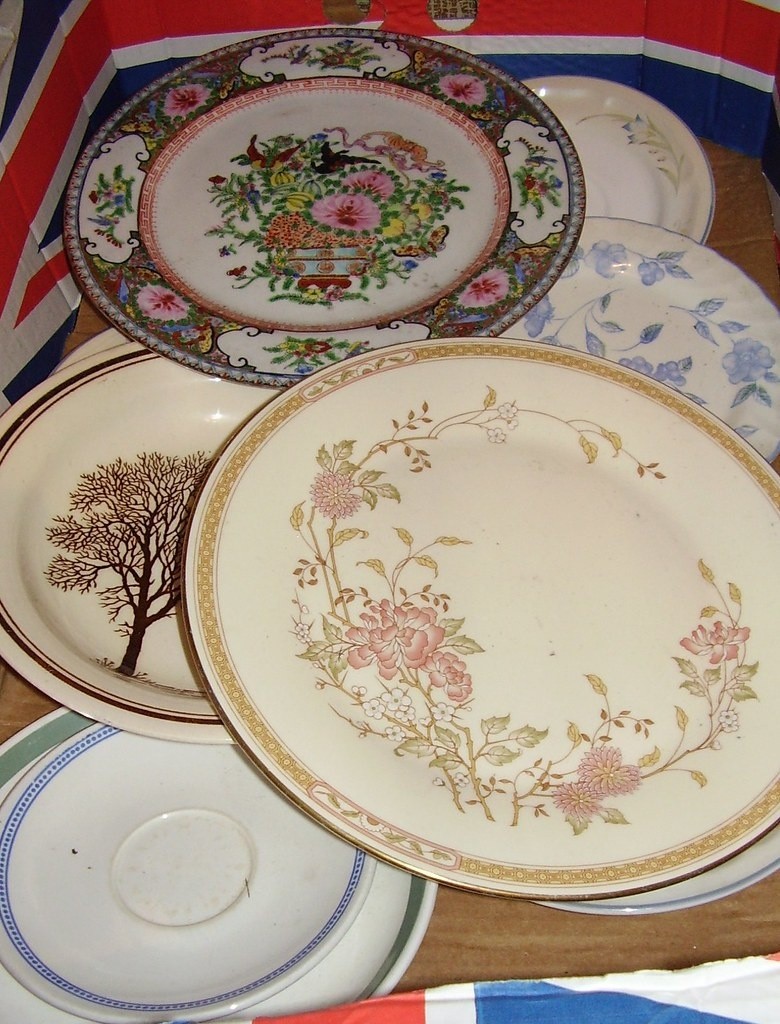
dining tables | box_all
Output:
[3,128,780,995]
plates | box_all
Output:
[61,26,581,386]
[499,218,779,469]
[515,73,715,250]
[0,340,288,744]
[1,707,439,1023]
[178,339,780,900]
[0,724,379,1023]
[534,822,779,918]
[48,324,134,377]
